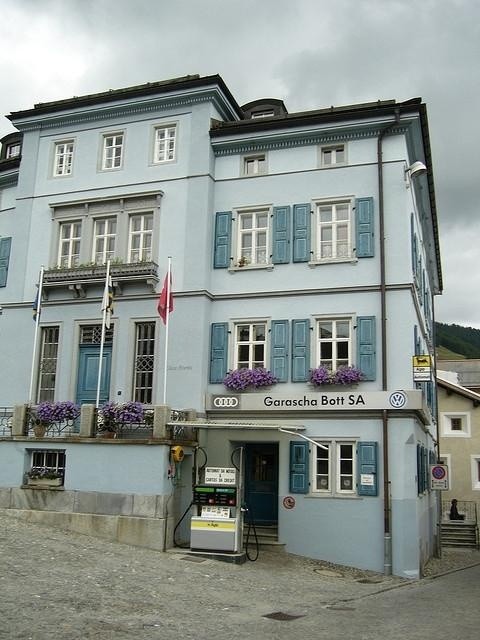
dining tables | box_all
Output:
[23,466,64,486]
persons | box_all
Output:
[449,498,465,520]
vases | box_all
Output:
[98,402,147,423]
[308,365,362,386]
[222,366,279,390]
[36,401,81,421]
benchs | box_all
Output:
[32,285,42,322]
[158,272,174,323]
[102,276,115,330]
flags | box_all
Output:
[102,430,118,438]
[32,425,46,437]
